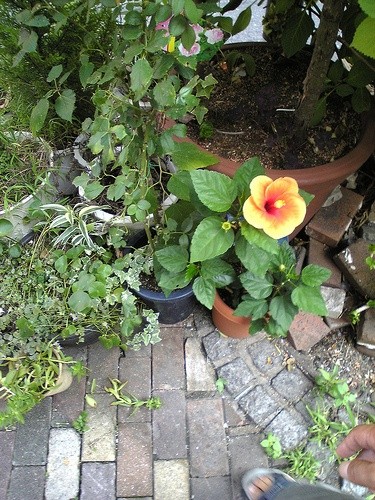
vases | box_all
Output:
[107,63,191,113]
[206,255,273,339]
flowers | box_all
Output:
[212,160,333,338]
[125,0,228,94]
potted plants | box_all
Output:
[76,61,186,236]
[147,0,375,252]
[0,98,58,239]
[0,335,74,433]
[109,159,204,324]
[1,221,125,350]
[76,108,136,168]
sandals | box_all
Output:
[240,467,294,500]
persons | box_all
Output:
[238,423,375,500]
